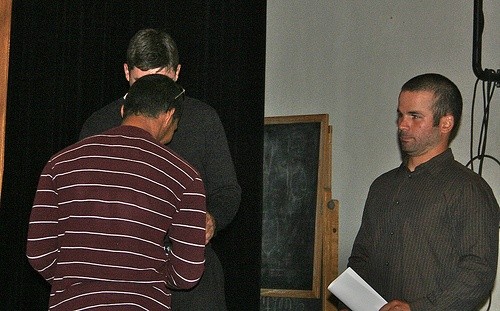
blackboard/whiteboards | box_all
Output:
[258,261,323,311]
[259,114,330,297]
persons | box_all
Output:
[346,73,500,311]
[79,28,240,311]
[25,75,206,311]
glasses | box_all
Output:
[122,86,186,113]
